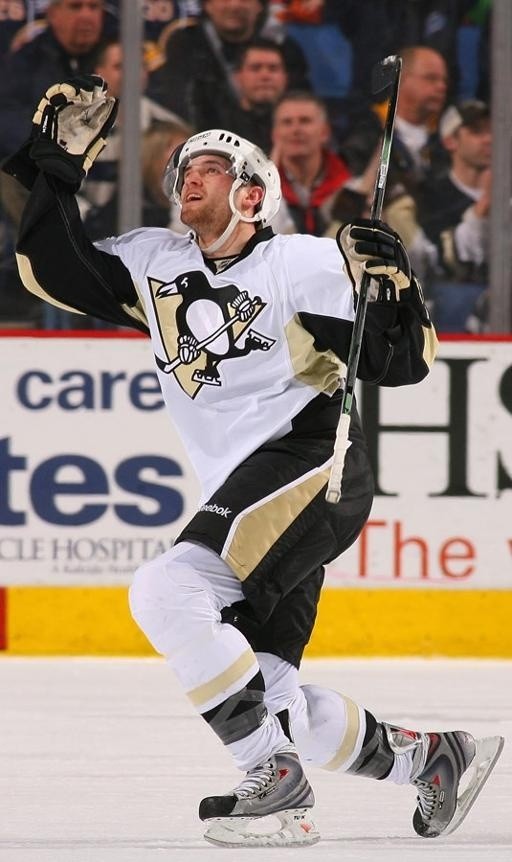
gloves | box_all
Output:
[336,217,432,337]
[0,73,121,193]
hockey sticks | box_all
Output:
[325,54,403,505]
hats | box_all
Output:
[161,130,284,231]
[438,98,488,140]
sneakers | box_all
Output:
[199,709,315,819]
[381,721,477,839]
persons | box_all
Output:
[2,2,496,337]
[9,71,479,841]
